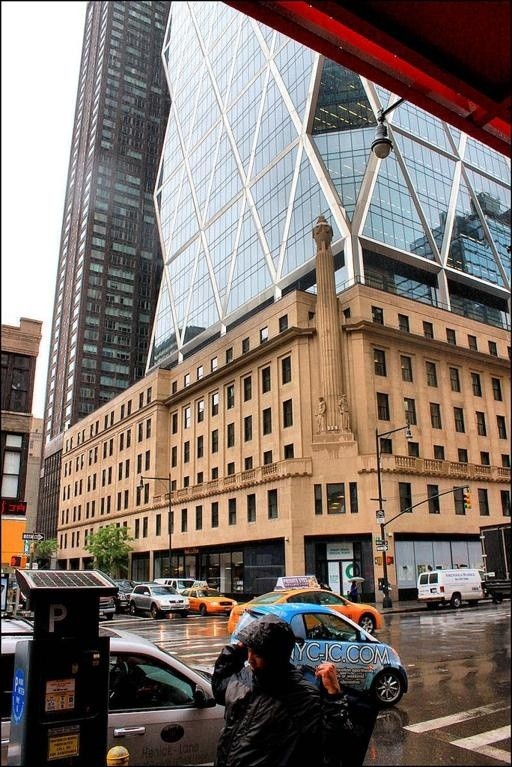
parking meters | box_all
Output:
[7,569,119,767]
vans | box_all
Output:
[416,568,492,609]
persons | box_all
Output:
[211,616,354,767]
[338,394,352,432]
[351,581,360,602]
[315,398,326,433]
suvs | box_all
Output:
[0,610,227,767]
[130,584,191,620]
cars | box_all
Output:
[230,603,409,705]
[209,564,284,592]
[8,572,197,610]
[227,575,383,636]
[99,596,117,619]
[181,580,238,615]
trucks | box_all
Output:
[479,520,511,600]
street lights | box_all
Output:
[138,472,174,576]
[373,422,412,608]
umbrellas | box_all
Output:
[347,577,365,583]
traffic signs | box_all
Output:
[22,531,46,541]
[376,546,388,550]
[376,539,388,545]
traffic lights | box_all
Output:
[387,557,394,565]
[462,492,472,510]
[9,556,21,567]
